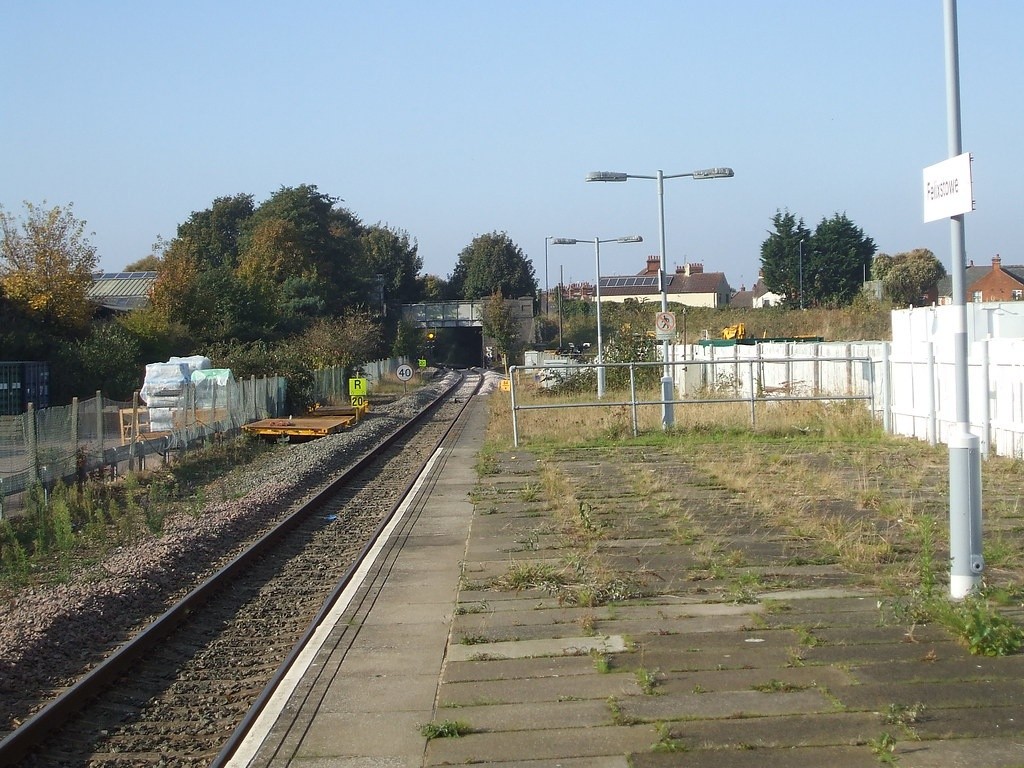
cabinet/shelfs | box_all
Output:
[119,408,149,446]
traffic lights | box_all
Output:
[428,326,436,339]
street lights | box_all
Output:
[800,238,805,308]
[545,237,553,317]
[550,235,643,398]
[584,167,735,431]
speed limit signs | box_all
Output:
[397,363,413,380]
